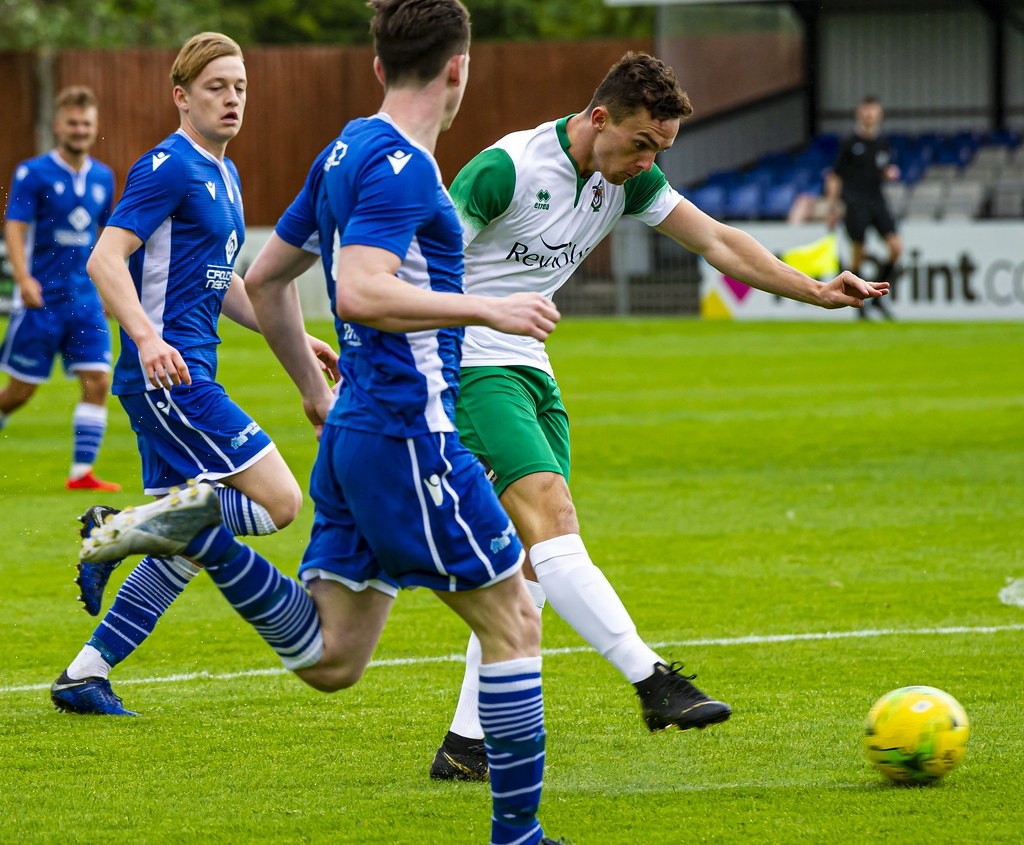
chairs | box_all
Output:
[675,130,1023,222]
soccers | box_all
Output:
[864,686,970,785]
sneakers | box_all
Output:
[79,479,222,564]
[73,505,128,615]
[65,470,122,493]
[49,669,139,715]
[632,663,732,733]
[429,745,490,782]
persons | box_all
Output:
[80,2,576,845]
[427,48,890,787]
[51,28,344,717]
[0,87,117,489]
[824,94,904,322]
